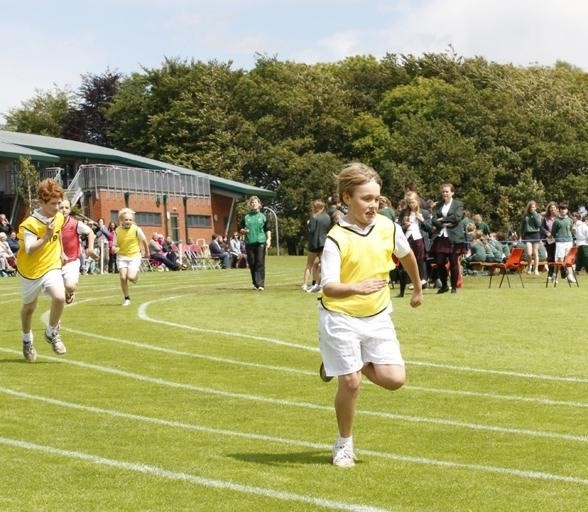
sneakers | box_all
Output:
[44,331,67,354]
[123,299,130,306]
[301,264,576,298]
[23,341,36,363]
[332,443,357,467]
[66,293,75,304]
[320,362,333,381]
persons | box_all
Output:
[59,198,96,306]
[1,213,18,278]
[318,163,422,469]
[74,217,247,275]
[16,178,67,362]
[378,182,588,299]
[302,197,349,294]
[238,197,272,291]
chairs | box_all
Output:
[546,245,581,288]
[0,240,247,278]
[488,247,525,288]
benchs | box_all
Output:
[470,262,548,272]
